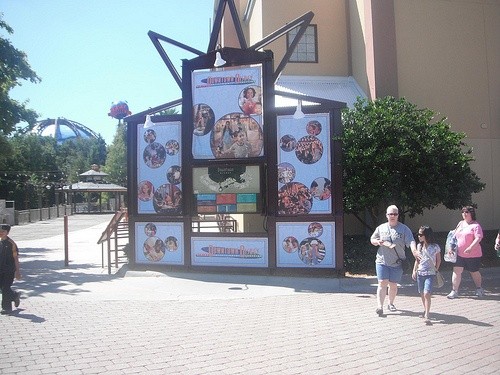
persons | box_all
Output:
[282,122,322,164]
[194,104,215,136]
[139,184,151,201]
[239,87,256,115]
[284,224,325,266]
[370,204,423,315]
[278,168,331,216]
[172,169,180,185]
[446,206,485,299]
[494,232,500,250]
[146,225,176,262]
[412,225,442,324]
[154,190,183,215]
[0,223,20,314]
[220,116,249,158]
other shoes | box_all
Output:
[388,304,396,312]
[1,307,12,314]
[14,293,19,307]
[376,307,383,316]
[419,310,430,323]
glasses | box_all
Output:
[388,214,398,216]
[462,211,469,213]
[416,234,424,237]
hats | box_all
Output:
[311,181,318,188]
[310,239,319,245]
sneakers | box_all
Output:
[475,286,485,298]
[446,289,459,298]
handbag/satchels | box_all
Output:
[432,271,445,288]
[443,229,457,263]
[401,259,409,269]
